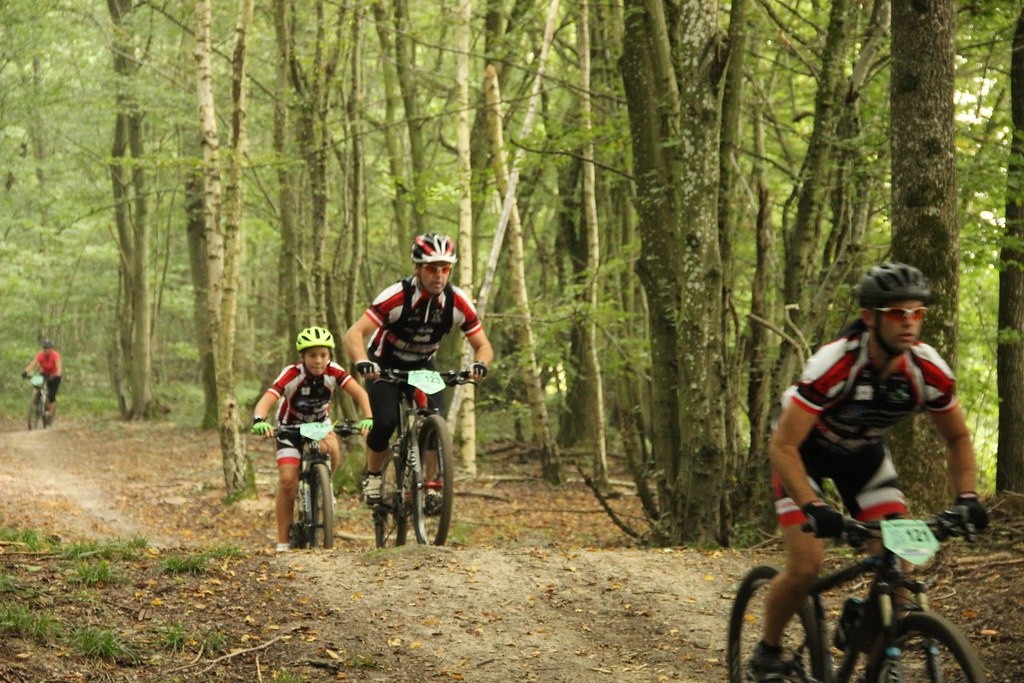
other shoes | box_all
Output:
[46,414,53,425]
[276,542,289,550]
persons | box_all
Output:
[252,327,374,553]
[344,232,494,516]
[752,261,991,683]
[22,341,62,425]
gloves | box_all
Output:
[953,490,990,543]
[21,372,27,378]
[802,498,845,540]
[54,376,59,382]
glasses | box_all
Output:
[870,306,930,320]
[420,265,451,275]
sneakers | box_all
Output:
[743,653,783,683]
[426,488,442,515]
[362,475,383,508]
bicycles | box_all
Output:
[20,375,58,431]
[264,418,361,552]
[348,369,479,547]
[728,505,988,683]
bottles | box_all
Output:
[832,598,863,653]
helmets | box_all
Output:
[296,326,336,352]
[411,232,457,265]
[43,339,52,348]
[855,262,932,308]
